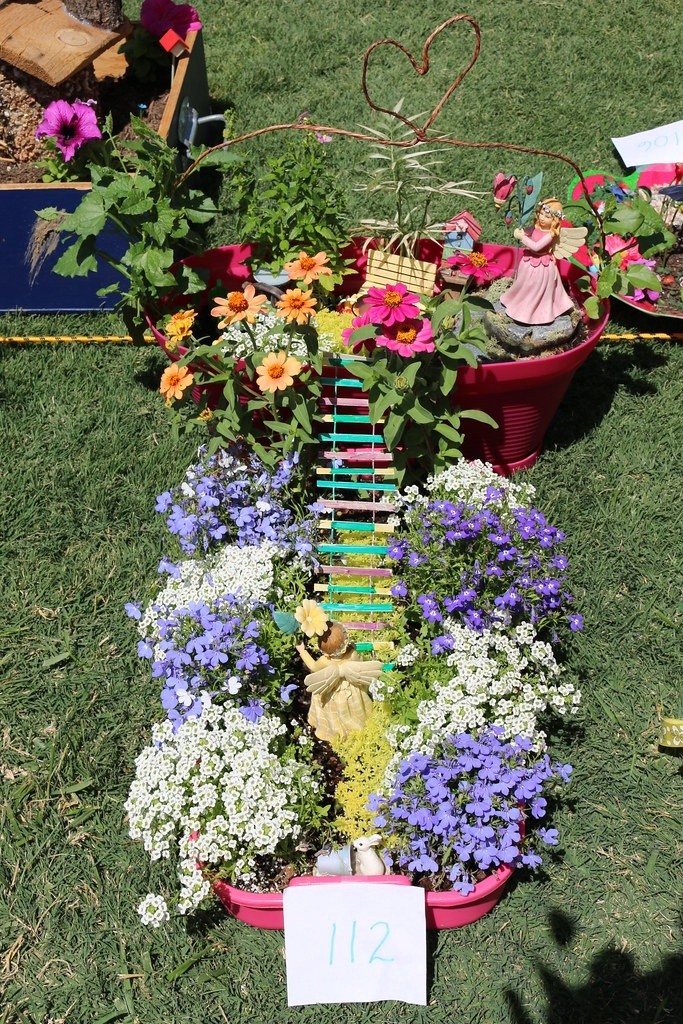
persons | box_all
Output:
[295,622,374,742]
[500,198,574,325]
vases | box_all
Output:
[188,795,524,929]
[0,16,204,313]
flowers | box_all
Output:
[36,81,682,925]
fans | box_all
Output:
[144,238,607,475]
